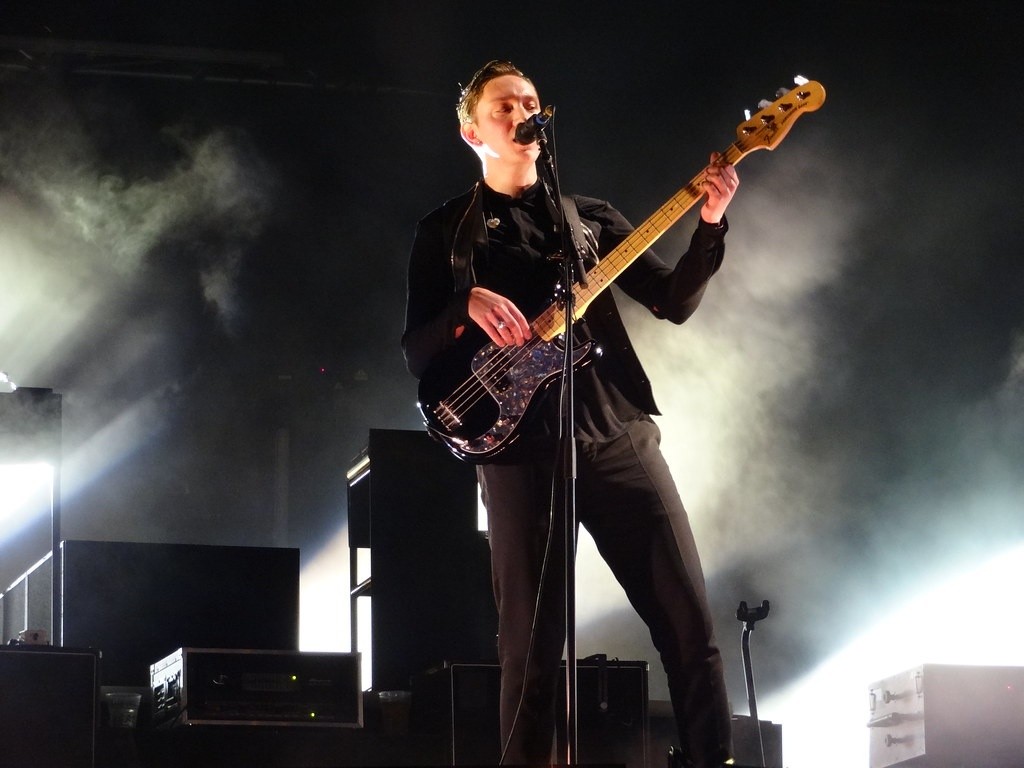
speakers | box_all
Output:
[409,658,651,768]
[0,644,101,768]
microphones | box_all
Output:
[515,104,557,145]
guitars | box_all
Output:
[416,76,825,464]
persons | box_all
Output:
[398,59,740,768]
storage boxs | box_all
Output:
[0,539,301,687]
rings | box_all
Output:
[498,321,505,328]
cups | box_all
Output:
[106,693,142,732]
